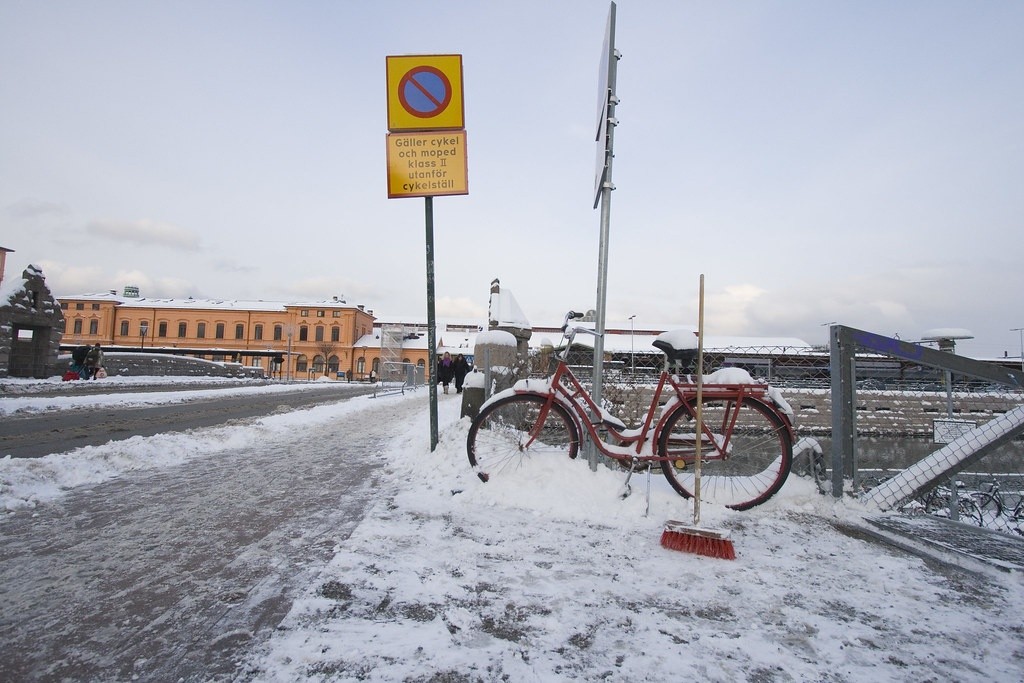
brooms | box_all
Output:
[659,273,737,559]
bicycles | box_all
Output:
[969,474,1023,535]
[865,473,984,527]
[467,311,796,511]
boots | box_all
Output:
[457,387,462,393]
[443,386,449,394]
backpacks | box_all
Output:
[73,346,84,360]
[87,350,98,366]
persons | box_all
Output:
[346,369,352,383]
[83,342,104,380]
[452,354,471,394]
[438,351,454,395]
[81,343,93,380]
[369,369,377,383]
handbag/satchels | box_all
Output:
[95,367,107,378]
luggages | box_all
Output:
[63,370,79,382]
